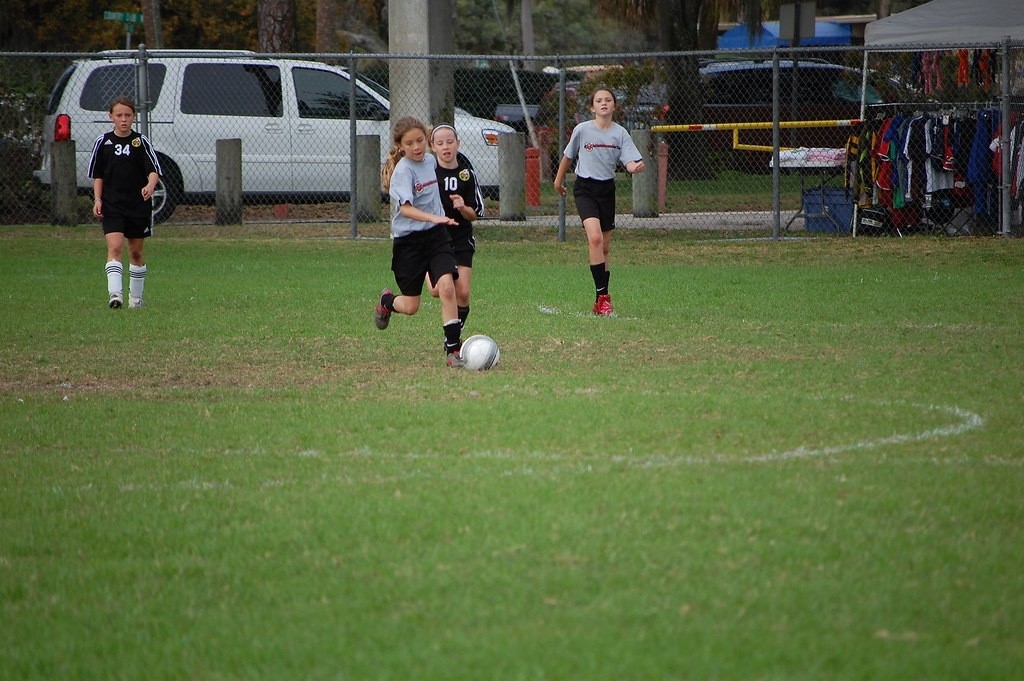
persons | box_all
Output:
[425,122,485,351]
[86,94,163,308]
[374,115,467,370]
[870,79,920,116]
[554,86,646,318]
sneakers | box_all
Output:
[108,295,123,309]
[128,293,143,309]
[375,289,392,330]
[594,294,614,315]
[445,351,464,367]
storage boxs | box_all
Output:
[803,188,857,235]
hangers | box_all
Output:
[874,100,1003,121]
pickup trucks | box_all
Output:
[491,79,667,171]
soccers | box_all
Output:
[459,335,498,370]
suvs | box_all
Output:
[653,54,942,180]
[28,49,520,227]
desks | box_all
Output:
[768,147,848,236]
